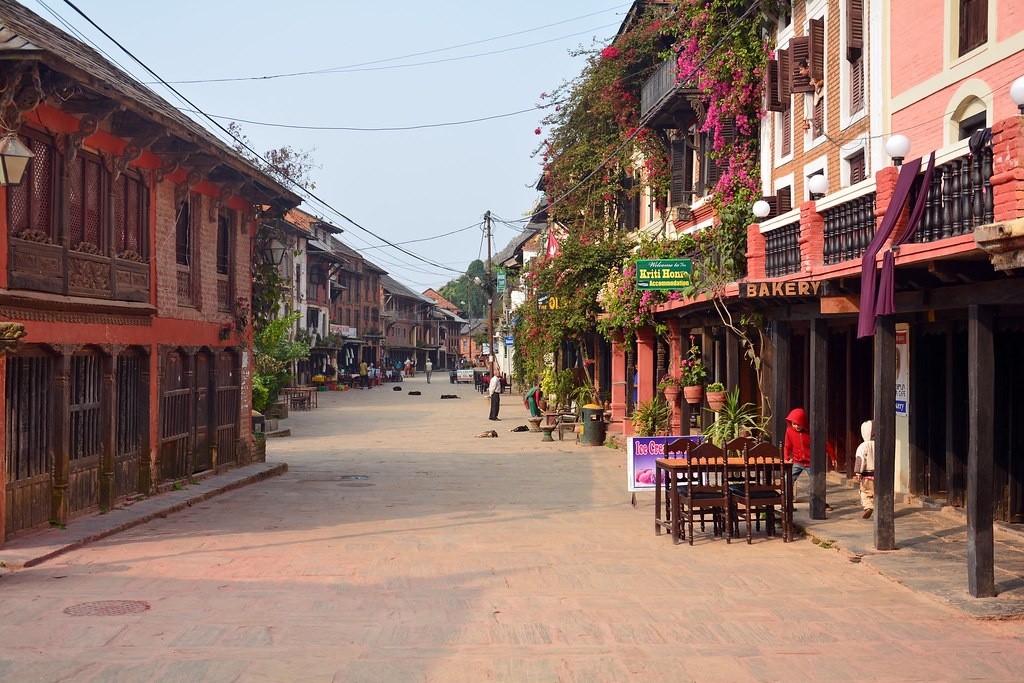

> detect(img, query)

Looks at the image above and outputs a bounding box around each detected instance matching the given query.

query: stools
[559,422,579,440]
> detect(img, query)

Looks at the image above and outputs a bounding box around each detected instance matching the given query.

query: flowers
[656,335,707,392]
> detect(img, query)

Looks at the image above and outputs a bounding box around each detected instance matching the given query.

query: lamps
[886,135,910,166]
[677,181,703,222]
[0,133,34,187]
[1011,77,1024,114]
[254,235,285,265]
[753,200,770,222]
[809,175,828,199]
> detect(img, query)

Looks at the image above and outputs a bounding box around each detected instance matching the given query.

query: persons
[360,357,416,389]
[633,361,638,411]
[489,369,501,421]
[423,358,433,384]
[784,408,837,511]
[854,420,874,519]
[524,383,542,417]
[463,356,467,365]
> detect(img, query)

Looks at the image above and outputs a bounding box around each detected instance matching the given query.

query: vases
[684,385,702,403]
[664,386,681,400]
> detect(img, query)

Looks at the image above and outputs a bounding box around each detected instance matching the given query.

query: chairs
[664,437,787,546]
[474,370,512,393]
[290,387,310,411]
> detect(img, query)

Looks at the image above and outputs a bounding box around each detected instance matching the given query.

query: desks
[655,457,794,545]
[284,387,318,409]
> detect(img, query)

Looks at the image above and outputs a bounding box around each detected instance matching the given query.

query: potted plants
[706,383,728,410]
[326,335,343,348]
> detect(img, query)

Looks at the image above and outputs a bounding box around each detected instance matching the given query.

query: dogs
[392,386,401,391]
[441,395,462,399]
[473,430,498,439]
[408,391,421,395]
[511,424,529,432]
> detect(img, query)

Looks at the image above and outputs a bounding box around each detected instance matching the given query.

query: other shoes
[826,503,832,511]
[494,418,501,421]
[863,508,873,519]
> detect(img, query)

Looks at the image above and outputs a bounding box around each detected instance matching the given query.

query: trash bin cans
[581,404,603,445]
[252,415,266,433]
